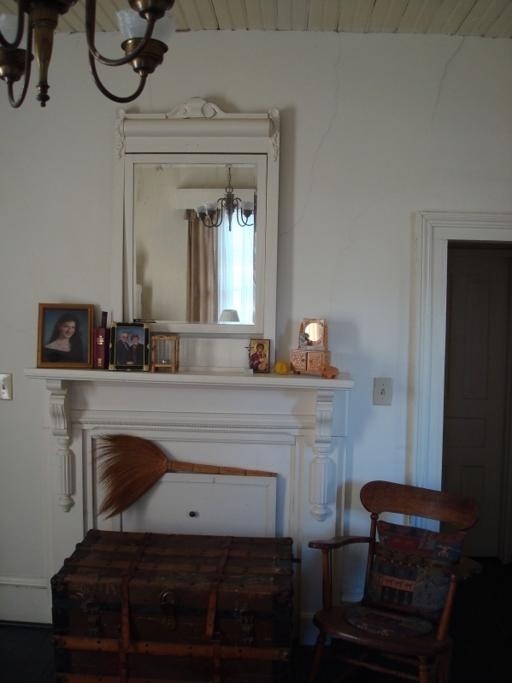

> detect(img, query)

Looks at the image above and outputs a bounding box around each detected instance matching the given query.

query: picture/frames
[150,333,179,373]
[108,320,151,373]
[249,339,271,373]
[36,302,95,369]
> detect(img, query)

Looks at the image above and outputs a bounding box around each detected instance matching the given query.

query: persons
[42,308,89,364]
[250,341,269,373]
[115,328,143,364]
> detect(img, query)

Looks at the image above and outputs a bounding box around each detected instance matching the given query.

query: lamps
[194,163,255,232]
[0,0,176,109]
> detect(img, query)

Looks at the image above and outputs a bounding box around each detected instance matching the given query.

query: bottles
[93,311,110,369]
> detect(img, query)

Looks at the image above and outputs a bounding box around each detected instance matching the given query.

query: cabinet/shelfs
[289,347,330,376]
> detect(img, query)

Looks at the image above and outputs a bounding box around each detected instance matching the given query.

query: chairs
[307,480,482,683]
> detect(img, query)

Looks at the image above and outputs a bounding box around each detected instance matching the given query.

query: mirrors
[298,318,327,350]
[106,98,282,369]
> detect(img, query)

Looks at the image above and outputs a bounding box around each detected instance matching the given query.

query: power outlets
[1,373,12,401]
[371,377,393,406]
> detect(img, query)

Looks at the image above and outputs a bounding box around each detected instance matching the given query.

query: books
[94,327,109,369]
